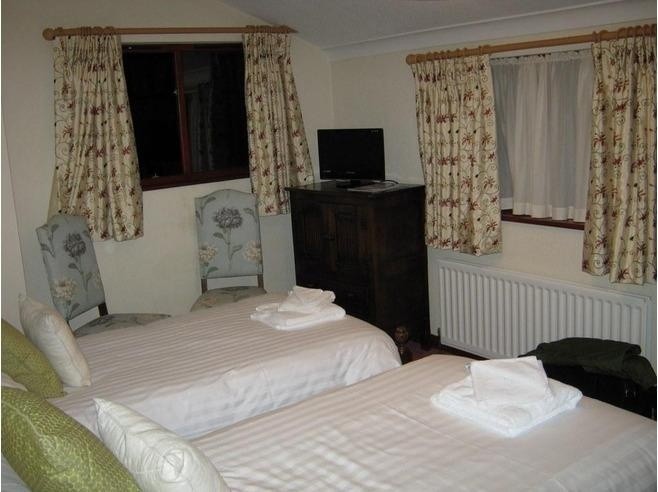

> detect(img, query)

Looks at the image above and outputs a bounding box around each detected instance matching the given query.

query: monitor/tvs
[317,128,385,188]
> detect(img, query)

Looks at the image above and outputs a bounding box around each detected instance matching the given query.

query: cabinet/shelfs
[283,178,429,356]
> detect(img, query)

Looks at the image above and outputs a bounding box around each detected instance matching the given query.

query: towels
[251,301,347,333]
[278,284,337,315]
[430,366,584,438]
[464,353,553,408]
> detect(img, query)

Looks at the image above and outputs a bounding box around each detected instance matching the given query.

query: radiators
[436,256,652,367]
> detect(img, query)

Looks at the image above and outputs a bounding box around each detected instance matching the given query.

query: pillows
[88,395,232,492]
[17,292,93,388]
[1,384,142,492]
[1,319,69,401]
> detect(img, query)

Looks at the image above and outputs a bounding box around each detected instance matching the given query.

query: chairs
[34,210,173,340]
[187,187,269,317]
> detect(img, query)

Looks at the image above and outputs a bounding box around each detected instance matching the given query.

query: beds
[1,353,656,492]
[1,283,404,455]
